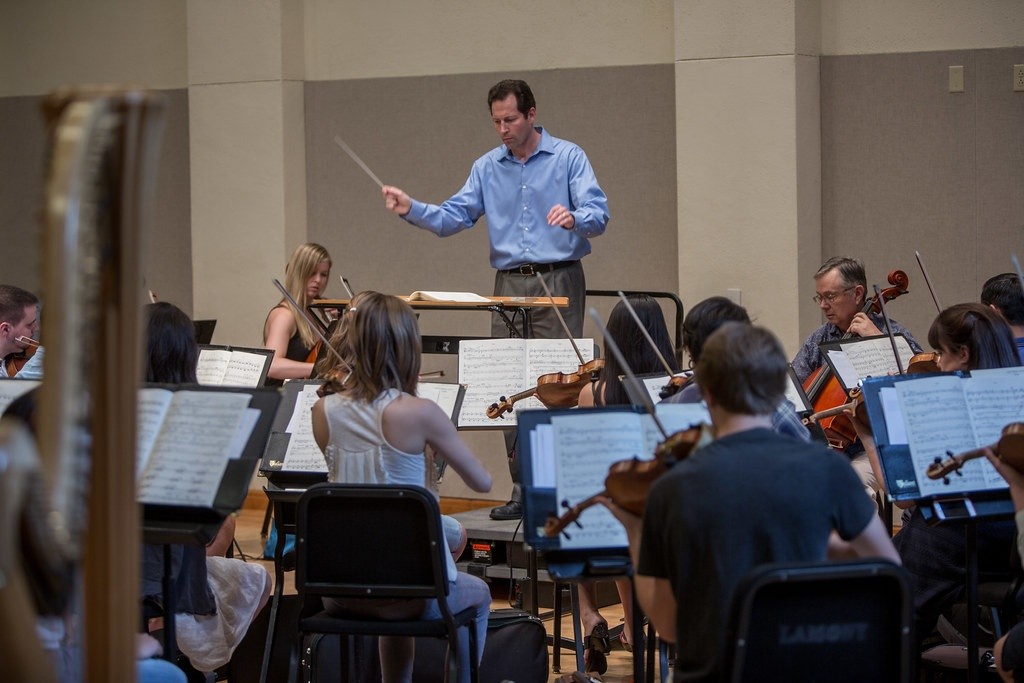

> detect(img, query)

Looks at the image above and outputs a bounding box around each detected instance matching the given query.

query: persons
[311,291,493,683]
[591,323,901,683]
[264,242,331,558]
[792,257,924,495]
[843,303,1021,644]
[0,285,273,682]
[980,273,1024,367]
[384,80,611,519]
[535,293,680,408]
[649,297,811,442]
[983,448,1024,683]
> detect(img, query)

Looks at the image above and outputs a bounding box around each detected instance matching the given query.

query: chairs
[286,482,482,683]
[663,556,921,682]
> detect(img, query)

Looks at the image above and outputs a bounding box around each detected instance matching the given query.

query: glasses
[812,288,856,306]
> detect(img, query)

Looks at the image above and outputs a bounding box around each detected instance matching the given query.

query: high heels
[620,626,647,653]
[583,623,611,675]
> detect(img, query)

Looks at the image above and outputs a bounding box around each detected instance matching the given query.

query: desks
[307,294,570,341]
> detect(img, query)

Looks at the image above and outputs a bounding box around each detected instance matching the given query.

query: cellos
[285,264,341,380]
[800,267,910,453]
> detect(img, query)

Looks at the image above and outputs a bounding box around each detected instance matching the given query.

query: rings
[563,214,566,218]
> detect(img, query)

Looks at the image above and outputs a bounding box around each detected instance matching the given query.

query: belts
[500,259,580,276]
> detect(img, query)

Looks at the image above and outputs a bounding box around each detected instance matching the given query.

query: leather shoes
[489,500,523,520]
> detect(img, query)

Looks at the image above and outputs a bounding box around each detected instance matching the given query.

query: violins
[926,421,1024,485]
[314,378,348,398]
[486,356,607,421]
[658,372,692,399]
[542,420,718,542]
[4,337,39,378]
[888,350,943,379]
[799,377,873,435]
